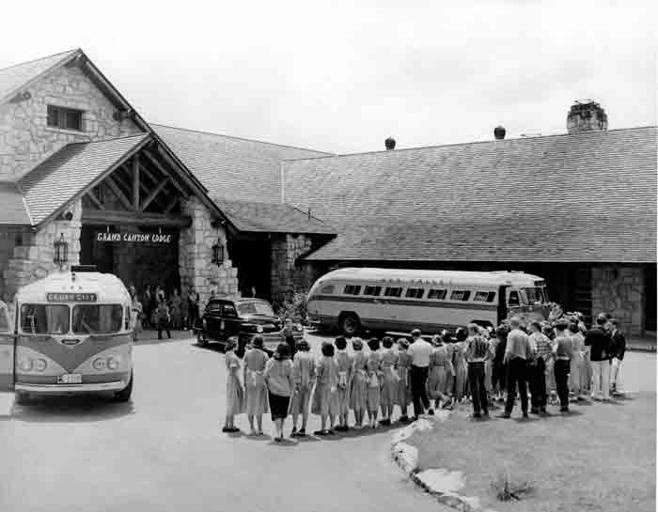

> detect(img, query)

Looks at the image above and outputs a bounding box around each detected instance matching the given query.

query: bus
[10,270,144,408]
[305,267,551,342]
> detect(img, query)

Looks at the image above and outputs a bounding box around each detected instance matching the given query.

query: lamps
[54,231,68,270]
[211,236,225,268]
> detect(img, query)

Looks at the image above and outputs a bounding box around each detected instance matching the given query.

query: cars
[191,294,305,359]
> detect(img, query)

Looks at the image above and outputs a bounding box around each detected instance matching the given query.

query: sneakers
[221,412,410,444]
[570,387,619,403]
[469,403,569,420]
[426,400,451,415]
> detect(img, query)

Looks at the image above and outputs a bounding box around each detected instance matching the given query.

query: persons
[223,318,436,445]
[429,312,624,423]
[128,286,200,340]
